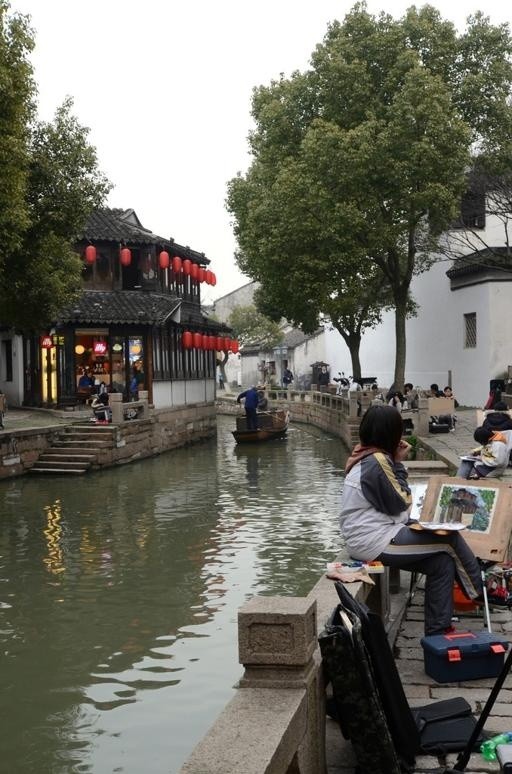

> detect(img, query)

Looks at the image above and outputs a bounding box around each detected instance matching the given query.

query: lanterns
[158,251,215,288]
[85,245,96,266]
[121,248,132,267]
[182,329,238,356]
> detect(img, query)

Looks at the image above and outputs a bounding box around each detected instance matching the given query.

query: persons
[317,364,330,392]
[218,371,226,389]
[340,405,512,636]
[257,391,270,411]
[454,426,510,479]
[282,366,293,389]
[79,368,113,425]
[483,401,512,467]
[237,386,260,431]
[347,377,459,433]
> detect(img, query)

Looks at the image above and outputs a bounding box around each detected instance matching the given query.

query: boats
[232,406,293,443]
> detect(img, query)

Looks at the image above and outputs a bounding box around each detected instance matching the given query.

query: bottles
[479,731,512,761]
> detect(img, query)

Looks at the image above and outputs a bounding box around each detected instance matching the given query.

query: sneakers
[470,592,509,610]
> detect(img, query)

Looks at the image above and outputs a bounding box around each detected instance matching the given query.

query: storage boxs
[418,628,512,682]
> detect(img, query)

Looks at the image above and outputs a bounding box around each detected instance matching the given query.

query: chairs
[328,580,492,772]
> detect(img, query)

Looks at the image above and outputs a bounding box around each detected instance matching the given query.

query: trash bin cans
[358,377,378,391]
